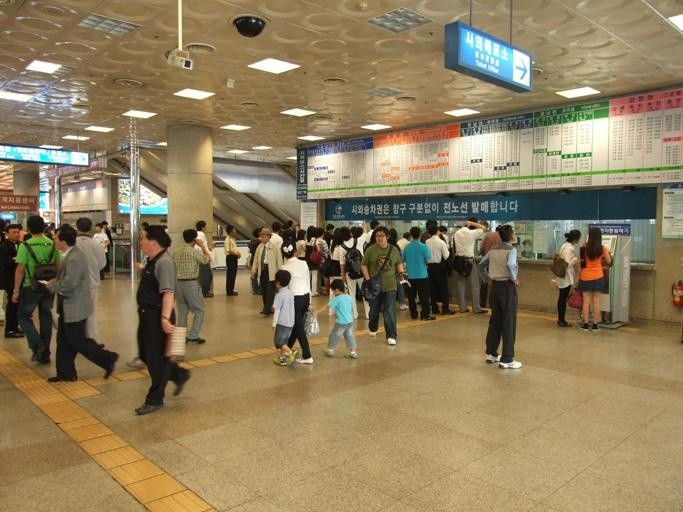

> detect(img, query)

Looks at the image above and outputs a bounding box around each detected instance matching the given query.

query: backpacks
[31,257,57,294]
[345,246,362,280]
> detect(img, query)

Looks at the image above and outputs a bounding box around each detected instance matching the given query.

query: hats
[257,228,272,235]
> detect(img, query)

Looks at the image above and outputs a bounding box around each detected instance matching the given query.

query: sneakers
[399,303,408,311]
[29,342,46,362]
[579,323,589,332]
[499,361,523,370]
[460,308,469,314]
[591,324,600,333]
[288,349,299,368]
[410,313,418,321]
[556,319,573,329]
[485,352,501,364]
[386,335,397,346]
[368,329,377,338]
[472,309,489,315]
[188,337,206,347]
[344,351,359,359]
[37,351,52,364]
[322,348,335,357]
[203,292,213,297]
[272,356,287,366]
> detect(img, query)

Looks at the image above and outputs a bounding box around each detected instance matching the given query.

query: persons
[553,230,581,327]
[247,216,503,321]
[224,226,241,296]
[578,228,612,331]
[0,214,118,383]
[363,226,406,346]
[478,224,524,370]
[126,222,150,371]
[172,228,212,344]
[314,279,360,359]
[195,220,214,299]
[136,226,191,415]
[279,238,314,364]
[272,270,298,366]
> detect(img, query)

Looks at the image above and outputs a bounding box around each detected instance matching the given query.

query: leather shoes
[442,309,455,316]
[296,357,314,364]
[48,372,77,384]
[131,402,165,414]
[420,313,434,321]
[227,291,238,296]
[103,351,120,380]
[3,327,24,339]
[173,369,193,395]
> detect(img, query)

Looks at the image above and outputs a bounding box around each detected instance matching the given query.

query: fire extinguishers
[671,280,683,308]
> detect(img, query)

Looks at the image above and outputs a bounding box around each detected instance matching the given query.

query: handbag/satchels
[452,255,474,278]
[310,250,322,265]
[566,288,583,310]
[361,273,384,303]
[550,254,567,279]
[302,307,319,338]
[320,259,340,278]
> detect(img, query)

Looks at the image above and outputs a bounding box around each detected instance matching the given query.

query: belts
[178,278,197,282]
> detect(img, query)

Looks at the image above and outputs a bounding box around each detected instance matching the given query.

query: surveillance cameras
[166,52,193,71]
[233,16,265,38]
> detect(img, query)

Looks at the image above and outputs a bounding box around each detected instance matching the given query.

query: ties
[260,246,266,271]
